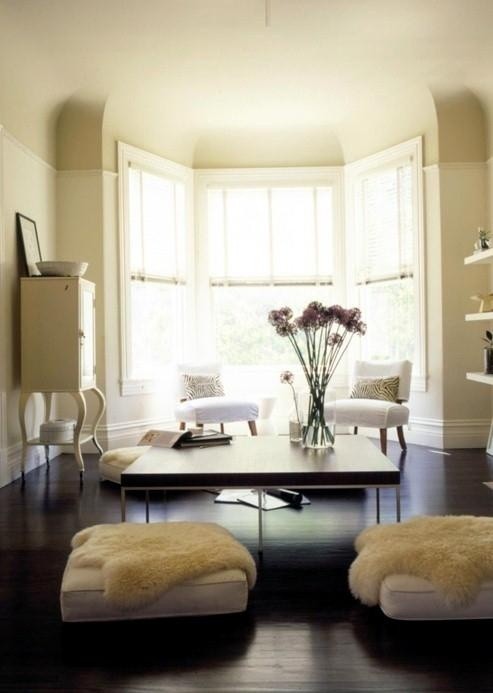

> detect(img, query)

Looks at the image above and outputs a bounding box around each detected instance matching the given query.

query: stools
[95,445,149,484]
[346,511,492,621]
[58,519,258,621]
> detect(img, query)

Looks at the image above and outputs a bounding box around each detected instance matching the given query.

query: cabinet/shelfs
[463,245,493,388]
[16,276,107,474]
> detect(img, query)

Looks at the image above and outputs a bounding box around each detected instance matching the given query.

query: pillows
[349,375,400,401]
[181,372,224,399]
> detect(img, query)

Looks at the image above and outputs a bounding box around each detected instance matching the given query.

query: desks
[117,433,403,527]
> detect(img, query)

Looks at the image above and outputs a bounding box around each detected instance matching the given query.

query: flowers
[266,299,367,445]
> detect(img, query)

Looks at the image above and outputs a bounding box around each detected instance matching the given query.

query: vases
[302,386,339,449]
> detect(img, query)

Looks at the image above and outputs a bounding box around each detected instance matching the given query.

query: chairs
[323,357,412,454]
[172,362,259,434]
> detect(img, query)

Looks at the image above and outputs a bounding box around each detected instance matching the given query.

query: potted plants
[480,329,493,375]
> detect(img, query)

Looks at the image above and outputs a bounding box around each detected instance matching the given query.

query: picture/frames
[15,210,42,276]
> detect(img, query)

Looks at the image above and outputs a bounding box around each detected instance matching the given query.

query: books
[214,489,311,511]
[136,429,232,448]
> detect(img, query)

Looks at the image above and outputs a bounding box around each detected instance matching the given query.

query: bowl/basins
[35,260,90,277]
